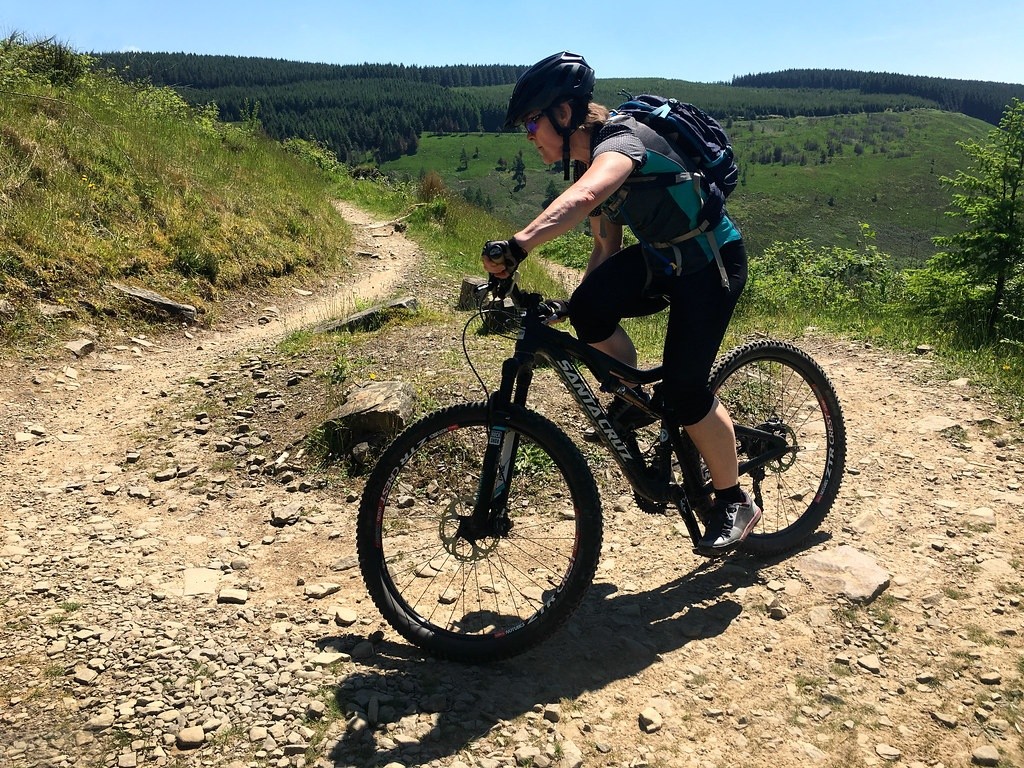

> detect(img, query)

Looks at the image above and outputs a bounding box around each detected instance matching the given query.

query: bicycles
[356,244,847,663]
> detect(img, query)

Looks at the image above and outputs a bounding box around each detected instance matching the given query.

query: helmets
[503,51,596,132]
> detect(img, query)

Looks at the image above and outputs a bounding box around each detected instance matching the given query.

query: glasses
[524,111,544,135]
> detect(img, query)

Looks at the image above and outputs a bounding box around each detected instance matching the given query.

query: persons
[481,51,761,555]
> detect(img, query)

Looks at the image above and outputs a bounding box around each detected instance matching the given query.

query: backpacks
[600,88,739,296]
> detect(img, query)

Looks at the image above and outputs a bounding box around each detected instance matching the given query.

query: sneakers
[697,492,762,551]
[582,386,650,444]
